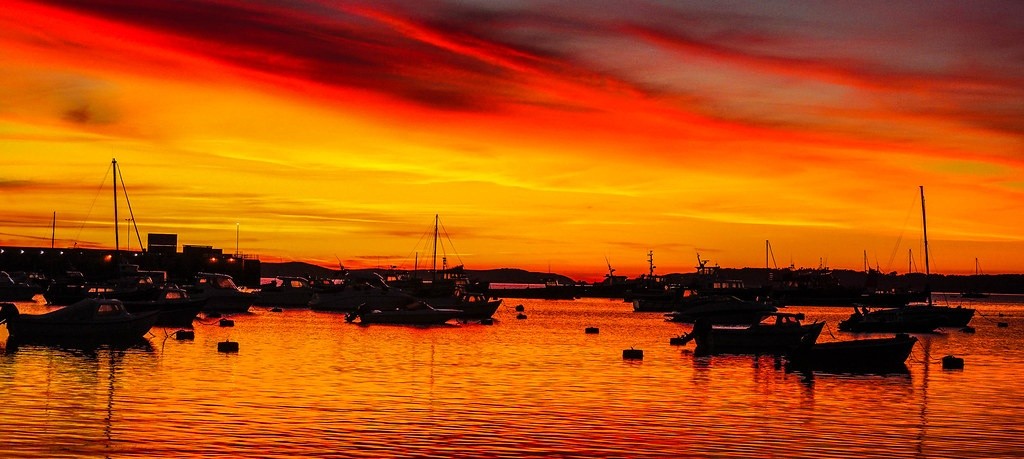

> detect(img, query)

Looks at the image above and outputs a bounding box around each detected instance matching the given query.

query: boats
[521,278,586,300]
[592,183,976,374]
[0,158,504,343]
